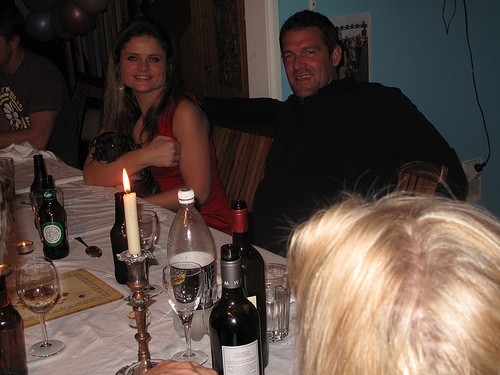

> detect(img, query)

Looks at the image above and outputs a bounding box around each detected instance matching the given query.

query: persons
[284,189,500,375]
[0,0,86,172]
[177,8,469,261]
[82,16,236,236]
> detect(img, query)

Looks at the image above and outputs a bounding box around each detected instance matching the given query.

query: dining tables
[1,143,301,374]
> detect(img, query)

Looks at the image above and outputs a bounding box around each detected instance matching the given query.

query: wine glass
[137,209,165,298]
[161,261,210,366]
[16,256,65,357]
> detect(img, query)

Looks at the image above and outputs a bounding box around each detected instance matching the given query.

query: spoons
[74,237,103,258]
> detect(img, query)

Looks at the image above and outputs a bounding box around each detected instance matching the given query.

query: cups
[264,261,290,343]
[0,156,16,203]
[125,358,165,375]
[29,186,64,244]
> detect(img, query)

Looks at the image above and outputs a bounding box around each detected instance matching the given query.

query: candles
[121,165,142,258]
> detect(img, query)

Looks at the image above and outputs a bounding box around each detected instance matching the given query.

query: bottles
[209,242,264,375]
[29,153,50,229]
[0,276,29,375]
[229,198,269,367]
[109,192,130,283]
[166,188,217,341]
[38,174,70,260]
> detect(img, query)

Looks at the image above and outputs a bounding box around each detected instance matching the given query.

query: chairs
[74,71,106,171]
[211,124,274,215]
[396,163,439,196]
